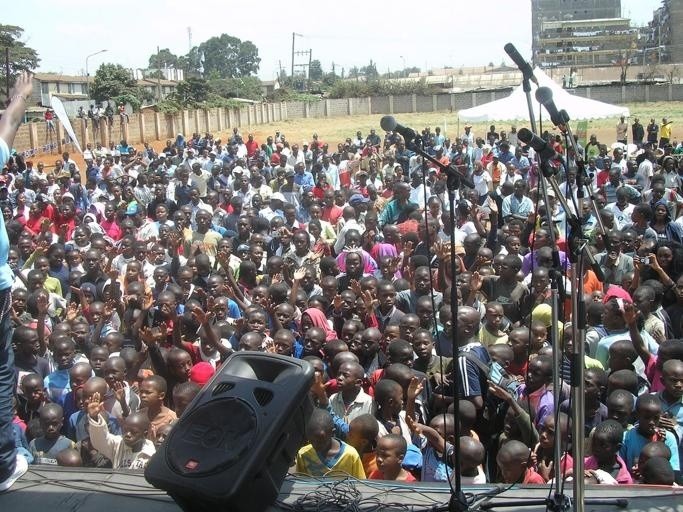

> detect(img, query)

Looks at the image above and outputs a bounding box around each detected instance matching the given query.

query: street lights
[85,49,108,101]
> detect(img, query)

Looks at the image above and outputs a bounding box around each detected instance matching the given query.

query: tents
[457,68,629,145]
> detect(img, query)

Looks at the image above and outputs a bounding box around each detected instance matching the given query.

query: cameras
[639,257,649,264]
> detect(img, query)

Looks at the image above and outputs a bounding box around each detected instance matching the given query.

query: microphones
[535,86,566,133]
[504,43,537,83]
[517,128,562,160]
[380,116,417,139]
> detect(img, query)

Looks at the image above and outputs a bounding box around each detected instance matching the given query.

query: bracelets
[12,95,26,102]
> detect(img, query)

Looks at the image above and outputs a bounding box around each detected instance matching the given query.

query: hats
[464,125,472,129]
[80,282,97,299]
[62,193,75,201]
[348,194,370,206]
[125,204,143,215]
[233,166,243,174]
[270,193,289,203]
[457,199,472,213]
[532,304,553,329]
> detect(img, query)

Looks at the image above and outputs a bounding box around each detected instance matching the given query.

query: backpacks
[458,342,517,434]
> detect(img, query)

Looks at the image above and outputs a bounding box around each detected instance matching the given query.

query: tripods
[415,189,505,512]
[480,281,627,512]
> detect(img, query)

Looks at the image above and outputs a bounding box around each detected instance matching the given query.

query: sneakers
[1,454,28,491]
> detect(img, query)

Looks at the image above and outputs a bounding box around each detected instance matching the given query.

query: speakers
[144,351,316,512]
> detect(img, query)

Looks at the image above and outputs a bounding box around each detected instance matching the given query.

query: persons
[45,101,129,133]
[0,128,683,485]
[1,69,34,491]
